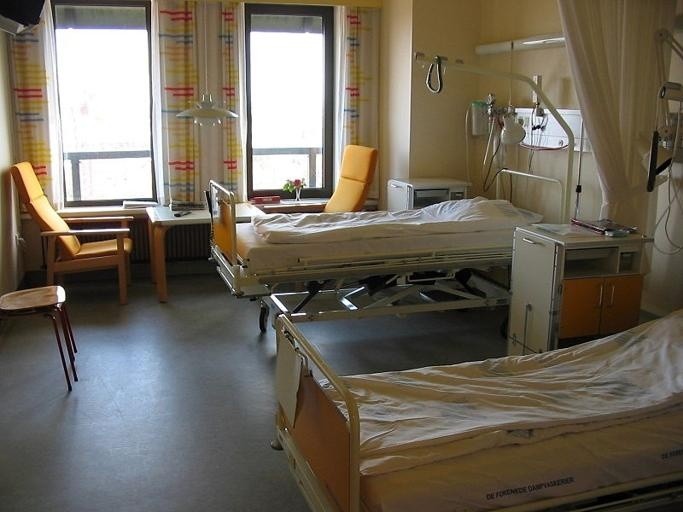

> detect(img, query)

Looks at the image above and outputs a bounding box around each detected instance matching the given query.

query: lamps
[175,0,239,129]
[500,111,526,147]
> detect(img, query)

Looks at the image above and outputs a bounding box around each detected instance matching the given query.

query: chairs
[323,145,377,212]
[11,162,136,305]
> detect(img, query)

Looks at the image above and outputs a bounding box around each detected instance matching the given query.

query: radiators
[40,222,211,268]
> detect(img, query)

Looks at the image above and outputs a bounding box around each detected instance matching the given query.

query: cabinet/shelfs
[507,223,655,356]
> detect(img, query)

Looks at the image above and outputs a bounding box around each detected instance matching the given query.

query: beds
[207,168,563,322]
[272,311,683,511]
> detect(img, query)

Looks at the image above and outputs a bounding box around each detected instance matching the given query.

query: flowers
[283,175,307,192]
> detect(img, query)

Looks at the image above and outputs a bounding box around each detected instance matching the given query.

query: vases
[296,188,301,201]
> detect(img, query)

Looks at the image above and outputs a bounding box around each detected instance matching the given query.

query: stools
[0,285,78,392]
